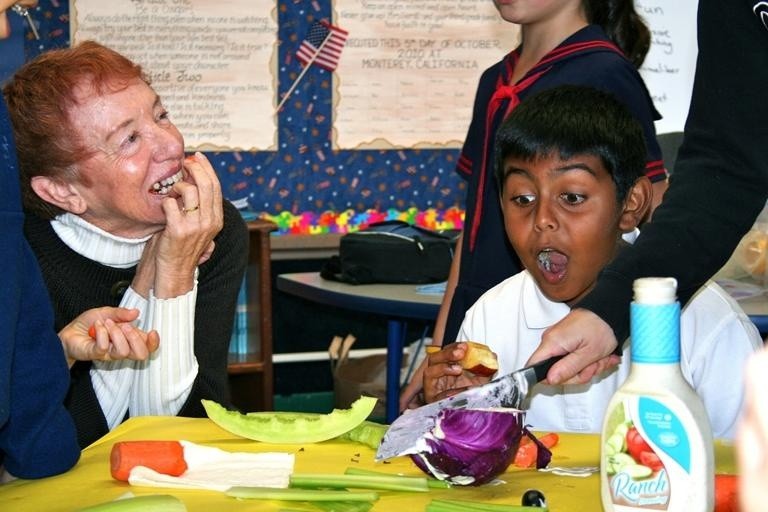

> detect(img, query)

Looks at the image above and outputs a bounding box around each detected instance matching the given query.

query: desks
[277,272,451,423]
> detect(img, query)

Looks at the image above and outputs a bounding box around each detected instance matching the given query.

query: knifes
[370,346,624,465]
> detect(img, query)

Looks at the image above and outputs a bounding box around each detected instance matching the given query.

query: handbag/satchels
[321,327,420,416]
[317,218,456,286]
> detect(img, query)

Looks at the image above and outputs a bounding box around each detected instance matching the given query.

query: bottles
[597,274,716,512]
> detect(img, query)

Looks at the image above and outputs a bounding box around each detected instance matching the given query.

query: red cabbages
[404,406,553,487]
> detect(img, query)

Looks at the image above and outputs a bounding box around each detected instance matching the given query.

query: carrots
[110,440,188,483]
[514,431,559,468]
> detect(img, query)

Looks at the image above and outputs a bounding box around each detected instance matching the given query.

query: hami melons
[201,394,378,443]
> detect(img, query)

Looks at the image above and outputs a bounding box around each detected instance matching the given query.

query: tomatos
[627,428,663,472]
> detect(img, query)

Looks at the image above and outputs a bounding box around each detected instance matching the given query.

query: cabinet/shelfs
[225,221,277,412]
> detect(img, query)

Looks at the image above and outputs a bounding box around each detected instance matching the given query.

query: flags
[294,18,351,75]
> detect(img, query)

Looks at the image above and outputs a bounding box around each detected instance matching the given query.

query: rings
[181,204,199,215]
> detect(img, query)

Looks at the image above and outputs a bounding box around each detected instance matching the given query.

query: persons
[0,1,162,490]
[0,39,253,454]
[521,1,767,389]
[393,1,676,415]
[417,83,768,448]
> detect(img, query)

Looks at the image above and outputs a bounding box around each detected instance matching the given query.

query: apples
[458,341,499,377]
